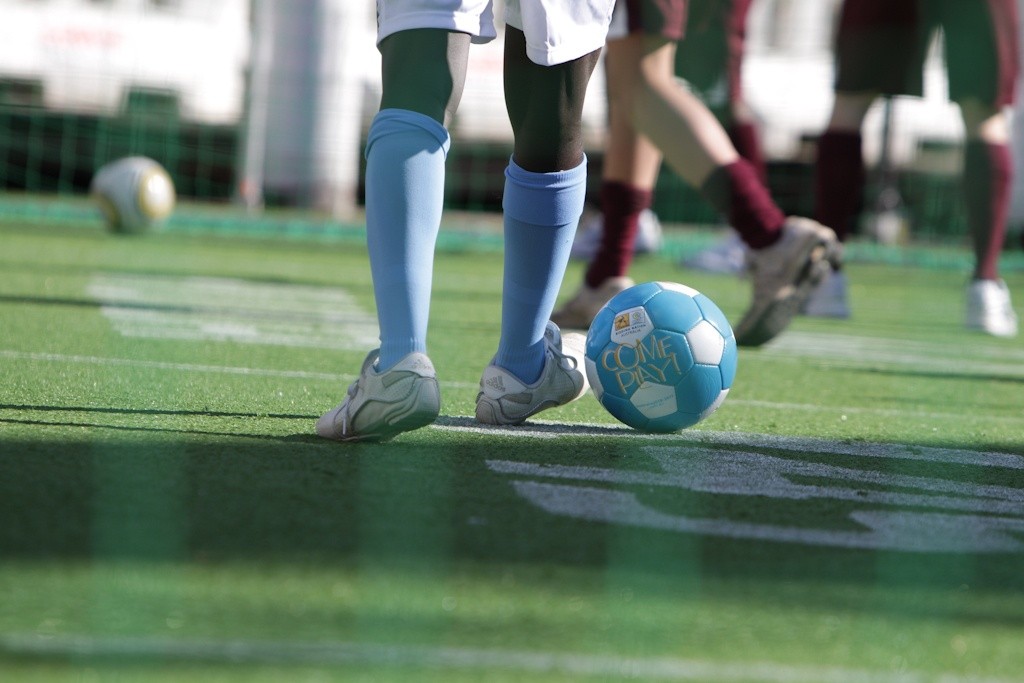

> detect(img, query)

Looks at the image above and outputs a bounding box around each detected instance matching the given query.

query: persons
[315,0,591,446]
[780,0,1024,341]
[548,0,835,349]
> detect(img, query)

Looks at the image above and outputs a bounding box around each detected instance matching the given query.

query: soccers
[584,278,738,434]
[92,157,175,234]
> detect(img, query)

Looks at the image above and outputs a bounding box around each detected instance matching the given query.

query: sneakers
[550,277,636,329]
[475,319,592,424]
[732,215,838,348]
[316,346,441,441]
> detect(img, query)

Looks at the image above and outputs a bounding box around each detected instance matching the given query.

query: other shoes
[681,229,751,278]
[964,278,1019,337]
[569,208,661,258]
[802,266,848,317]
